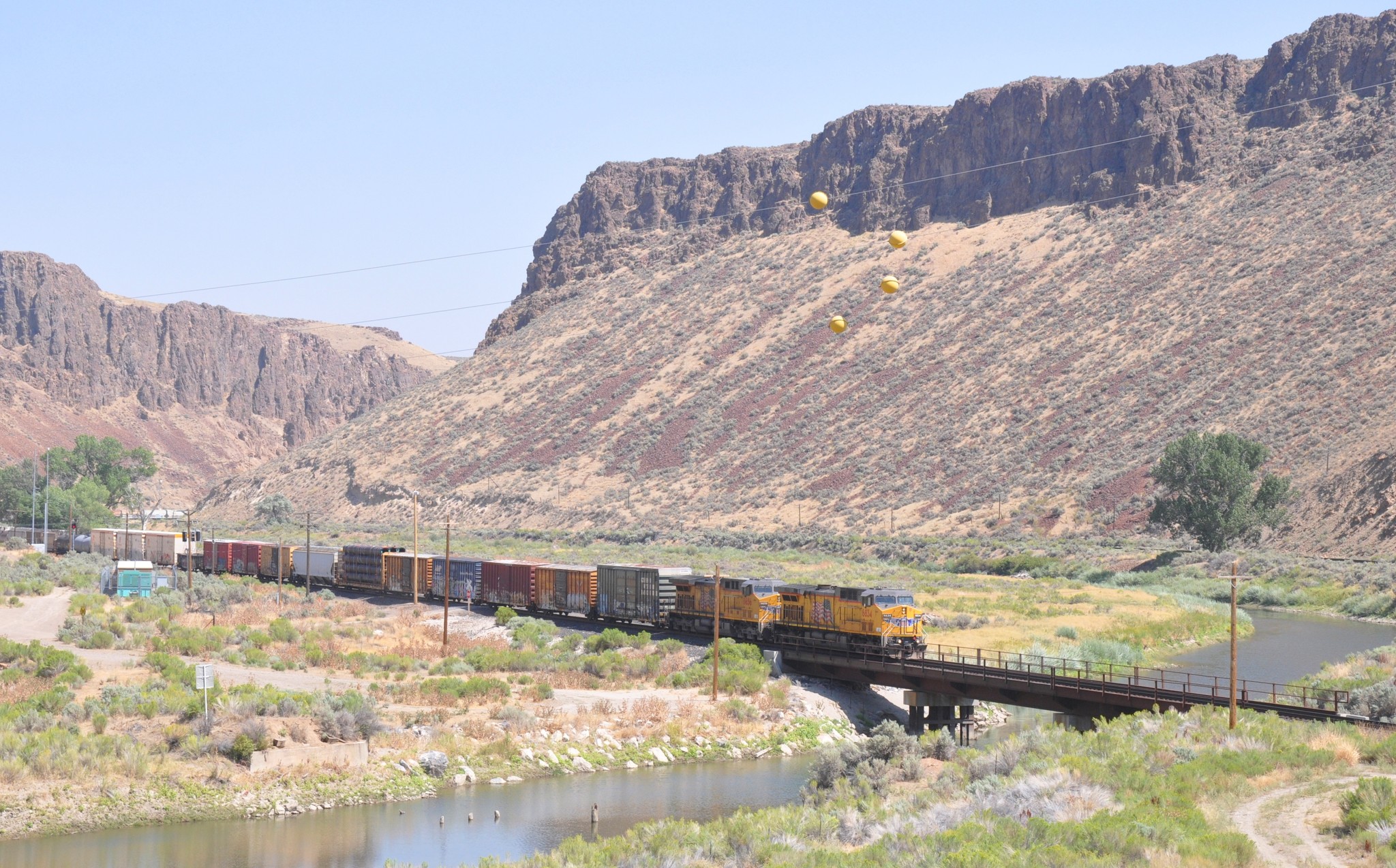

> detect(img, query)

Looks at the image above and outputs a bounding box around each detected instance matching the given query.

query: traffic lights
[71,523,76,529]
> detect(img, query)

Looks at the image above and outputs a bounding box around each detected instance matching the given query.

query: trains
[1,525,928,663]
